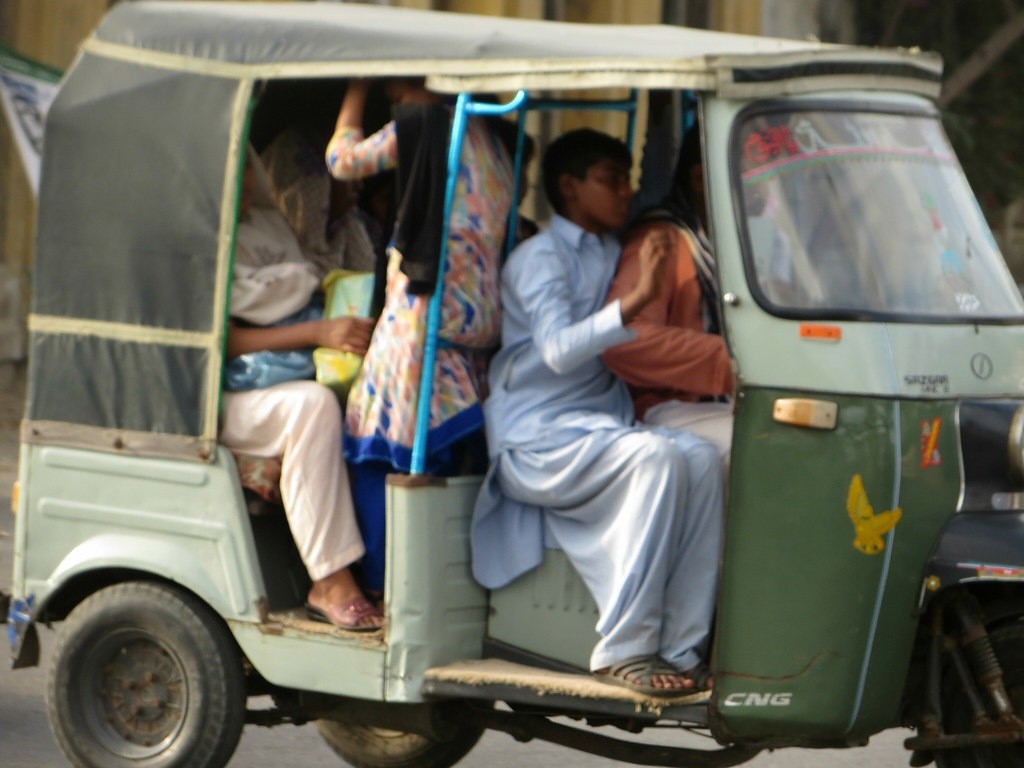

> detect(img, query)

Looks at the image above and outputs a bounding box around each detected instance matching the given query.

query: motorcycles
[8,0,1024,768]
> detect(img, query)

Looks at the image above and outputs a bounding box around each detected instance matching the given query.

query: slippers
[305,600,383,630]
[683,661,713,690]
[593,653,696,695]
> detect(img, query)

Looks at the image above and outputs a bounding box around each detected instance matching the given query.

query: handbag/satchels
[311,270,378,404]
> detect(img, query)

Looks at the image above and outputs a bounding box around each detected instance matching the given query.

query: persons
[216,76,538,630]
[469,117,767,693]
[959,196,1024,447]
